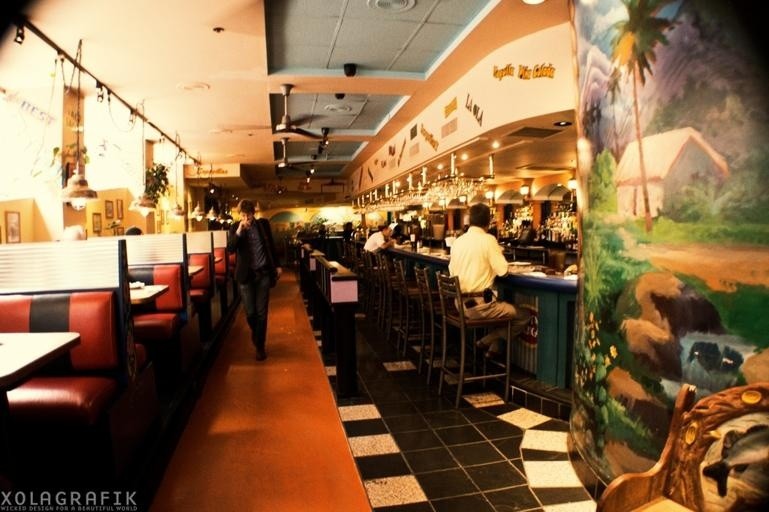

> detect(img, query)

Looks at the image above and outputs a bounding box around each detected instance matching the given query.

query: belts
[462,293,483,297]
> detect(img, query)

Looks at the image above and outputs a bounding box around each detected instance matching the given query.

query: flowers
[141,164,171,200]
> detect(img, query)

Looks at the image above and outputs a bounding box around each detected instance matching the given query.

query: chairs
[338,239,478,384]
[435,271,515,409]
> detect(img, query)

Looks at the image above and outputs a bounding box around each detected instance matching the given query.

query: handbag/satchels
[269,260,276,289]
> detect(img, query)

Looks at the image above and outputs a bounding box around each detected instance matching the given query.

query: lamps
[129,100,158,217]
[168,132,236,225]
[53,38,100,212]
[347,150,578,209]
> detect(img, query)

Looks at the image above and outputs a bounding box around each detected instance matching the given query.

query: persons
[126,225,144,235]
[228,198,282,360]
[448,204,532,359]
[343,222,355,241]
[360,226,398,258]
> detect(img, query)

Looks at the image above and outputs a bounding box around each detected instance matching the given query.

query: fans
[221,83,327,140]
[252,137,318,173]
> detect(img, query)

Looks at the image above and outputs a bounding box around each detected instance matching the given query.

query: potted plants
[140,193,156,202]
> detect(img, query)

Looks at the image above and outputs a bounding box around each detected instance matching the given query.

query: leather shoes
[484,351,506,362]
[255,350,266,361]
[477,342,487,352]
